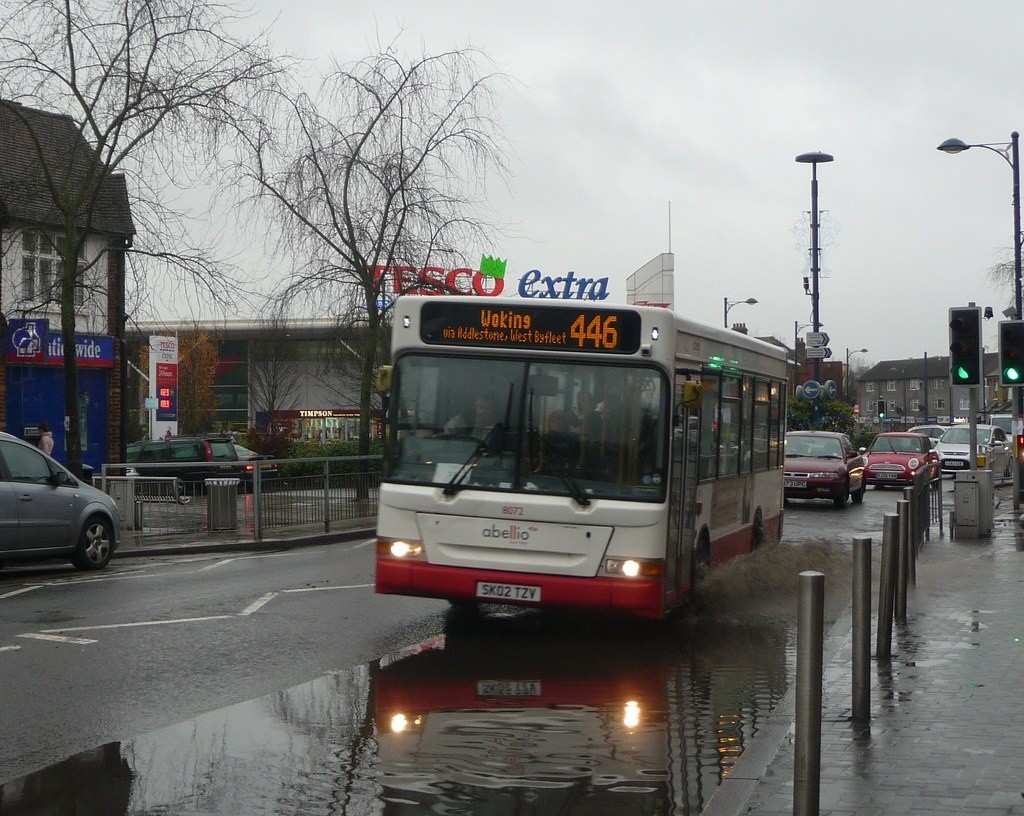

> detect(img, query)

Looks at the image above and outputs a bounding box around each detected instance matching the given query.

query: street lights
[847,348,868,401]
[794,152,834,420]
[937,138,1022,511]
[794,323,824,398]
[724,297,759,328]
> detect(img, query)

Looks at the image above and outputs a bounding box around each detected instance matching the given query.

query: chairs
[908,439,920,449]
[822,441,839,455]
[809,446,824,457]
[791,441,808,455]
[976,433,987,443]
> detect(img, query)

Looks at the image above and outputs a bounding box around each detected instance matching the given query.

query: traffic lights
[947,306,983,388]
[877,400,884,418]
[998,320,1024,387]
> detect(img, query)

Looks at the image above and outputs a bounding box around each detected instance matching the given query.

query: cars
[859,432,941,490]
[0,431,120,572]
[989,414,1013,442]
[933,424,1013,481]
[908,424,949,449]
[233,444,282,494]
[784,432,868,510]
[126,436,240,504]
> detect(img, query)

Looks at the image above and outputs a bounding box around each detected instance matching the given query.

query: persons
[429,390,516,460]
[549,389,659,482]
[220,430,239,444]
[37,422,55,457]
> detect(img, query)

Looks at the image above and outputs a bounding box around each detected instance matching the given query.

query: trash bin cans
[203,478,240,530]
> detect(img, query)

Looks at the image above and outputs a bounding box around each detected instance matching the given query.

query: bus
[375,293,789,617]
